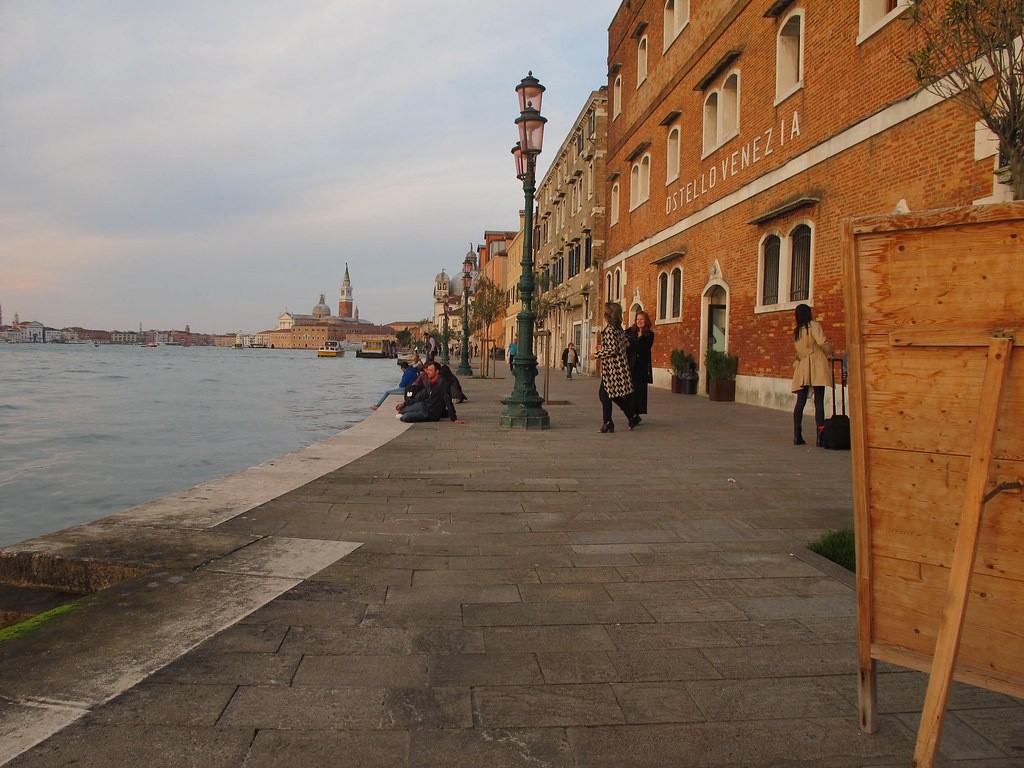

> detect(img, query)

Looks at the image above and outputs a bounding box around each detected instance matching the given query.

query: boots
[816,430,821,447]
[794,429,805,445]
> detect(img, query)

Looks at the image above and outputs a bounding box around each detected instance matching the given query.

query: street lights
[440,293,452,366]
[455,259,473,378]
[498,70,551,430]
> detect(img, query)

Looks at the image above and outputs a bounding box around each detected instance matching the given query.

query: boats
[317,340,345,358]
[249,344,269,348]
[140,342,158,347]
[356,339,399,359]
[397,346,418,366]
[232,343,243,350]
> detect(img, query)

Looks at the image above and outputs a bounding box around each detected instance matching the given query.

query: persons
[790,304,835,447]
[561,342,581,380]
[370,333,496,424]
[507,336,518,372]
[624,311,655,425]
[590,302,641,433]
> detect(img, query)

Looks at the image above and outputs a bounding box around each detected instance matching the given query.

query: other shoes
[567,376,572,380]
[395,414,403,420]
[370,405,378,410]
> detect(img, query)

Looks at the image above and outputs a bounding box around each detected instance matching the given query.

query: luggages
[820,358,850,450]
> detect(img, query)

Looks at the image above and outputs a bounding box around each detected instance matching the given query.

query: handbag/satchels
[560,362,564,371]
[576,363,581,374]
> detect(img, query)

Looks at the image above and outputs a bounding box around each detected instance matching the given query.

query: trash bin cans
[679,362,696,394]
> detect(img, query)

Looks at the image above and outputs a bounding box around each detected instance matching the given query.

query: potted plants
[667,349,699,394]
[495,348,505,360]
[704,350,739,401]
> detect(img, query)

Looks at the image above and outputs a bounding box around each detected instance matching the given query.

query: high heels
[598,422,614,433]
[628,415,641,430]
